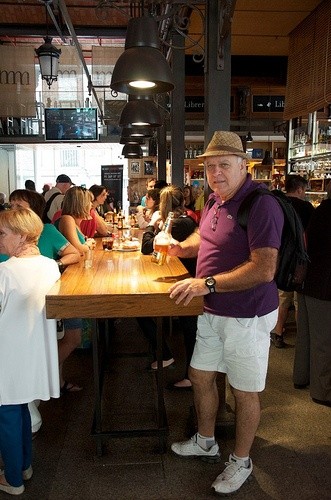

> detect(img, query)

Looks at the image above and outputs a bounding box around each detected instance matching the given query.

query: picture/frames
[128,159,157,178]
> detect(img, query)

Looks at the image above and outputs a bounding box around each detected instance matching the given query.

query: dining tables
[46,218,204,456]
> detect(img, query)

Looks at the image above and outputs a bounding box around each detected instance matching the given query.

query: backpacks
[238,190,307,293]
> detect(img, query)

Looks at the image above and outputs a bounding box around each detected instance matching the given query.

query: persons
[271,174,315,348]
[153,130,285,494]
[0,173,204,440]
[0,207,61,495]
[292,183,331,407]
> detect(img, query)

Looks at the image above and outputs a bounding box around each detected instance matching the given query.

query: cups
[78,249,93,269]
[131,228,138,237]
[101,237,114,251]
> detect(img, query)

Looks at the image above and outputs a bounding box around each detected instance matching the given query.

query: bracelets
[57,260,62,266]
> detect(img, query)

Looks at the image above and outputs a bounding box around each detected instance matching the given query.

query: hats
[196,130,248,162]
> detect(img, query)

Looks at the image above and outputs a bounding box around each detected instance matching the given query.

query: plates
[114,246,142,251]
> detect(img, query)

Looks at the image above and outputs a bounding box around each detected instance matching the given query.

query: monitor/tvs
[44,108,99,142]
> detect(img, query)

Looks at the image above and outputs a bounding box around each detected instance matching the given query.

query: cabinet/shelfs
[159,139,286,195]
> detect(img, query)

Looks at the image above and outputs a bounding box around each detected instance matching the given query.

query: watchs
[205,277,216,292]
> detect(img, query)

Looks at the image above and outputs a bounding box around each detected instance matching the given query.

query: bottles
[118,213,123,229]
[151,211,174,266]
[167,144,201,159]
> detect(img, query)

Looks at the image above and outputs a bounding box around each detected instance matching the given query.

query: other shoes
[312,398,331,407]
[167,380,192,391]
[294,382,310,388]
[60,379,87,392]
[271,333,285,348]
[151,355,173,369]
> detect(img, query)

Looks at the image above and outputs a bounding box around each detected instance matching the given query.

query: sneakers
[211,454,253,496]
[0,469,25,495]
[23,465,34,480]
[170,434,220,456]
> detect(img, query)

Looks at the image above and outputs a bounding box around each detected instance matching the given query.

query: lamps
[33,0,61,89]
[93,0,207,158]
